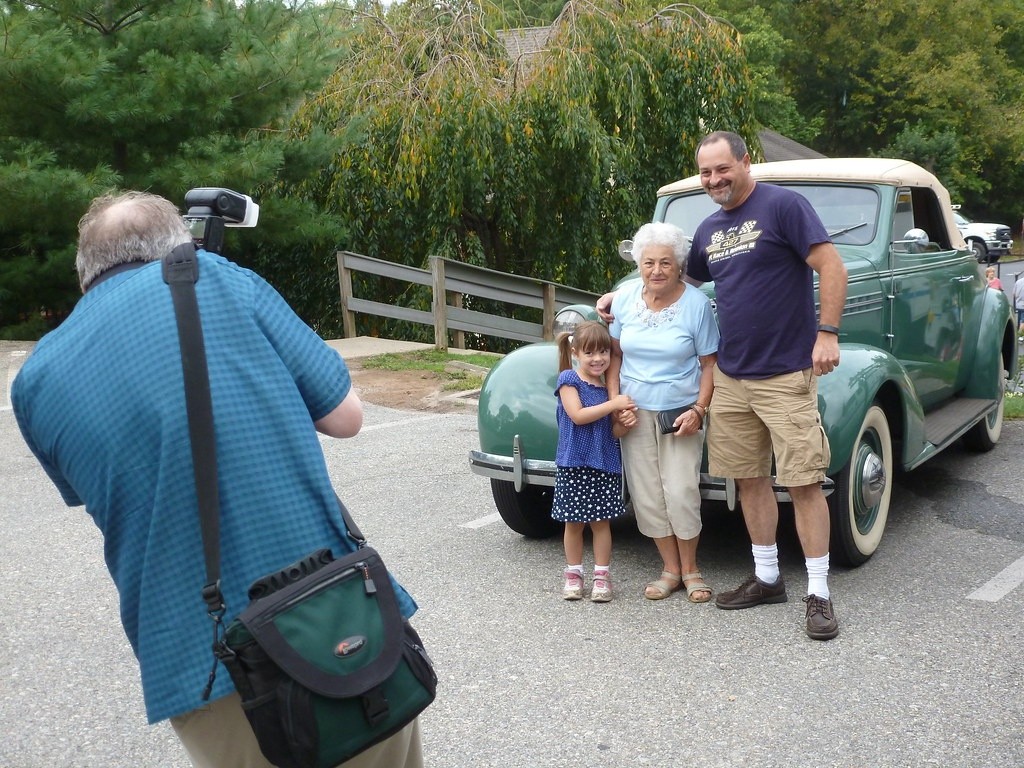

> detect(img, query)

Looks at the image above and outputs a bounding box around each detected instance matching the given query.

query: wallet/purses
[656,407,702,435]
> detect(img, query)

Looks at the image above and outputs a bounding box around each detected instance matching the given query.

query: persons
[985,267,1003,293]
[552,323,638,602]
[1012,273,1023,341]
[606,222,720,605]
[12,185,424,768]
[597,132,846,641]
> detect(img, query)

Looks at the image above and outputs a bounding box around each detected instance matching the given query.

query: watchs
[695,403,710,414]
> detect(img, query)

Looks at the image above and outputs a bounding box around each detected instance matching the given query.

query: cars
[468,157,1018,571]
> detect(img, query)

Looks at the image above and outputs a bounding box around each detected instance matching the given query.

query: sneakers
[591,570,613,602]
[563,569,584,600]
[805,595,839,641]
[714,575,788,610]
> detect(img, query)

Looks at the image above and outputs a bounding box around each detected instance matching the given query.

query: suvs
[945,207,1012,264]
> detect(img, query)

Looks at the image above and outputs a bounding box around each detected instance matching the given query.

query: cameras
[181,186,260,258]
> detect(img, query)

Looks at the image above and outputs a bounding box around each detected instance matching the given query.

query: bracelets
[817,324,840,337]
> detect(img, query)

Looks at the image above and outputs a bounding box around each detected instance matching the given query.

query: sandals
[644,571,682,600]
[682,572,713,603]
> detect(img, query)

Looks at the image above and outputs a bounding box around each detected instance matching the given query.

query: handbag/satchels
[219,547,439,768]
